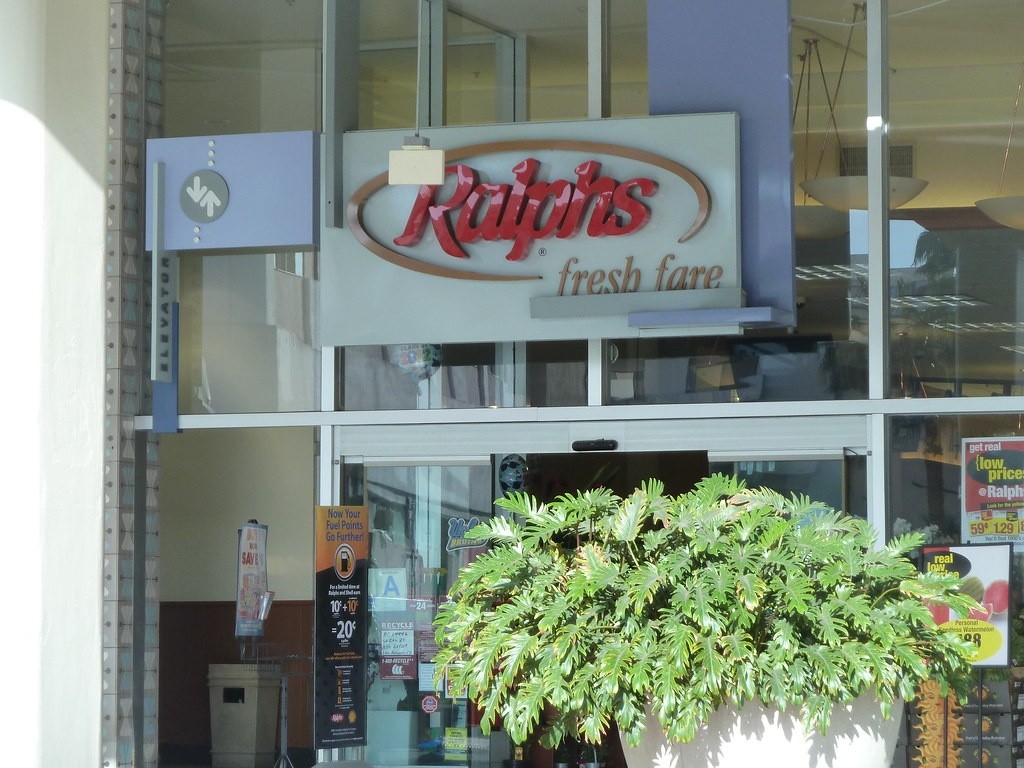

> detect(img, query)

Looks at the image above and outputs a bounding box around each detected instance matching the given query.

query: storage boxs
[905,667,1024,768]
[444,727,468,761]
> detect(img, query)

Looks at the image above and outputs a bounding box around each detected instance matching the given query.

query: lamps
[387,0,444,186]
[975,62,1024,231]
[793,38,849,241]
[798,0,929,214]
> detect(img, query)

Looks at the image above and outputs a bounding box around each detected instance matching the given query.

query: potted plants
[430,473,990,768]
[554,741,572,768]
[583,739,608,768]
